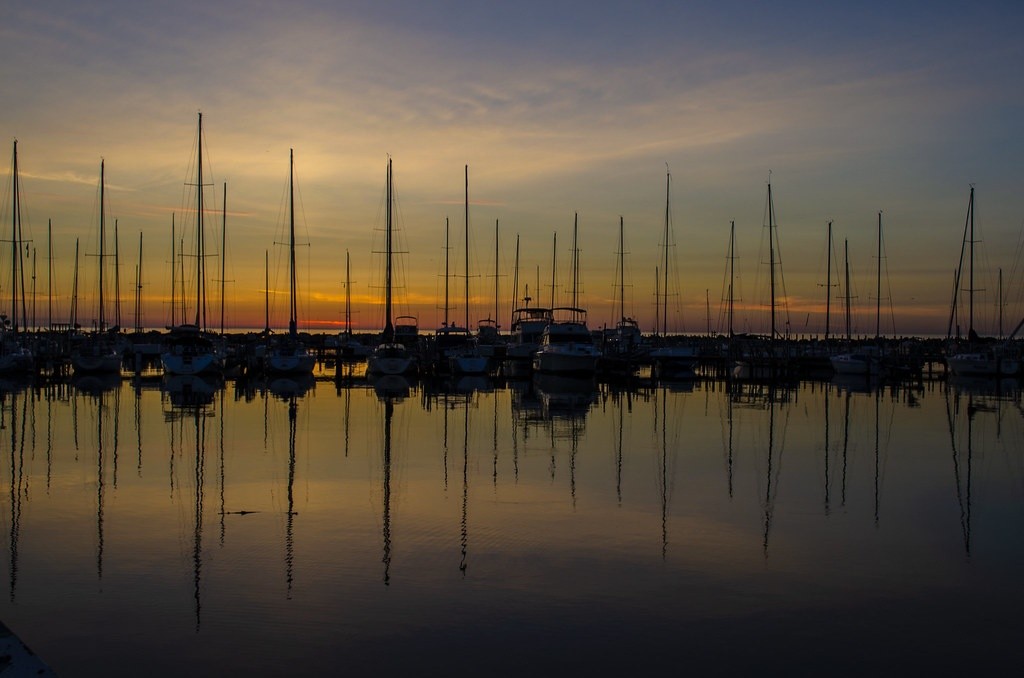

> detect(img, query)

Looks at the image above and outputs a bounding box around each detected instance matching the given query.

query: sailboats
[263,372,316,602]
[420,374,487,578]
[162,370,225,634]
[0,111,1024,379]
[366,368,418,583]
